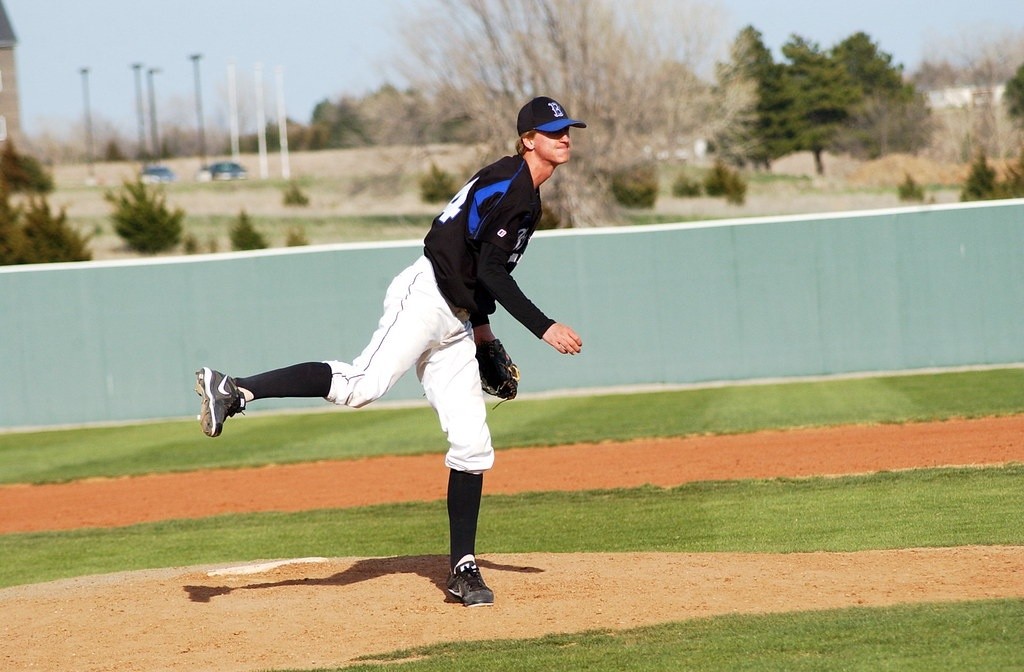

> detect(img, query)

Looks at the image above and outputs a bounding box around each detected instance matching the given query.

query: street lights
[79,68,93,177]
[143,69,160,165]
[131,62,150,167]
[190,55,208,169]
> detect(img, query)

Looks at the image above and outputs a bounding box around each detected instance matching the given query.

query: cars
[138,165,177,187]
[204,161,248,183]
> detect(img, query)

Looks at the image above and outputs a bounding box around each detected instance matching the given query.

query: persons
[193,97,587,608]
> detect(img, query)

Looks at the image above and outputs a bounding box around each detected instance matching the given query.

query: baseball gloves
[476,339,520,400]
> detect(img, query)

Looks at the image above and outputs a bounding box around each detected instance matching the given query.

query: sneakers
[445,565,493,608]
[196,366,248,438]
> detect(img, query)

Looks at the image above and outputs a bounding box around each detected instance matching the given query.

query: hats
[517,96,587,136]
